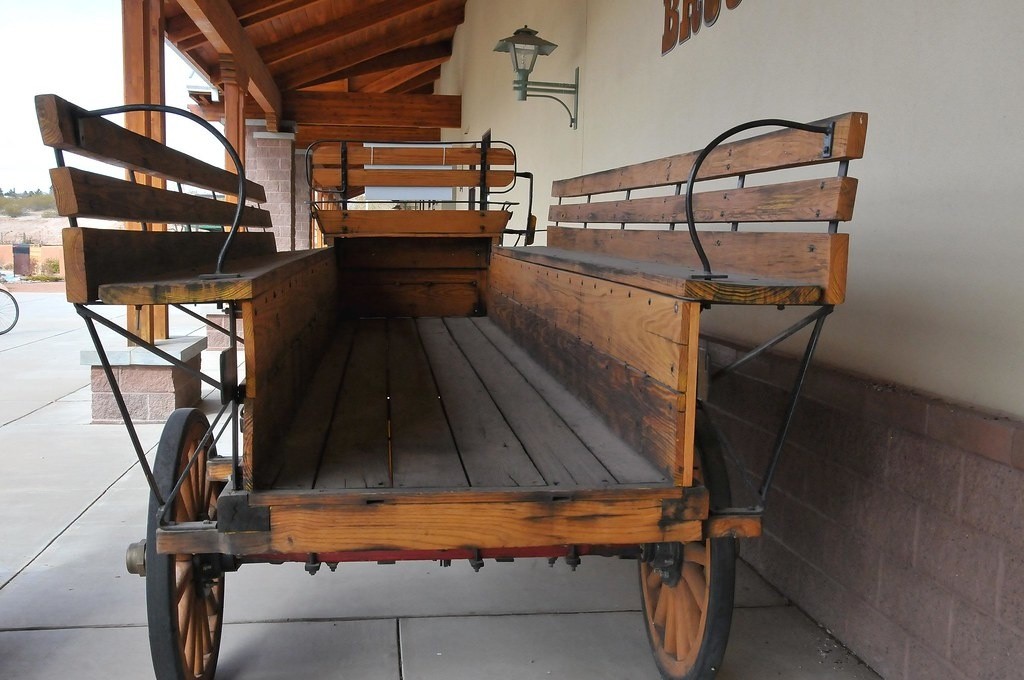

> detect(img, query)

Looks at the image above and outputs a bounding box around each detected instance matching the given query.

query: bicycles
[0,288,19,335]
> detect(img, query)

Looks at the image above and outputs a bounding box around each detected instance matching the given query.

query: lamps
[494,24,580,130]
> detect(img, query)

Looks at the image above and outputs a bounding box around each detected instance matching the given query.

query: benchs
[306,140,516,238]
[35,94,335,307]
[494,110,868,315]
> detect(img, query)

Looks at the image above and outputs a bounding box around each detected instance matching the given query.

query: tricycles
[35,95,870,680]
